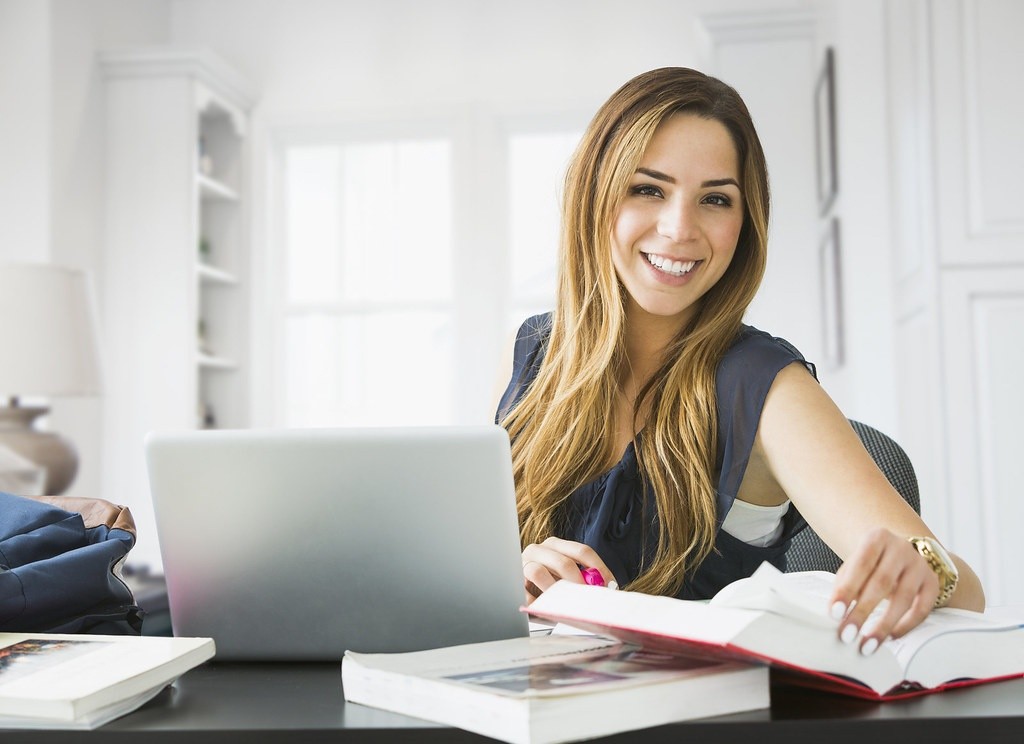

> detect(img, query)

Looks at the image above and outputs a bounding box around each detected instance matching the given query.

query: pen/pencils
[581,566,608,587]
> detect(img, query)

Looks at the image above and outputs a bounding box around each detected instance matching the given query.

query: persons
[496,67,985,657]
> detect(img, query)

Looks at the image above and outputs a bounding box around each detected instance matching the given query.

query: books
[520,559,1024,701]
[341,632,770,744]
[0,633,216,731]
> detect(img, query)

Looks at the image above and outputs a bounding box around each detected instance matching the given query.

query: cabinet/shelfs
[99,56,253,571]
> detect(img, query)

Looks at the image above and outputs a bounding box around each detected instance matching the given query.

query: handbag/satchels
[0,490,149,636]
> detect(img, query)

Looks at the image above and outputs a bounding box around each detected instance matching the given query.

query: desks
[0,612,1024,744]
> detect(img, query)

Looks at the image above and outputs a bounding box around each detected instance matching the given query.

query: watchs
[909,538,958,608]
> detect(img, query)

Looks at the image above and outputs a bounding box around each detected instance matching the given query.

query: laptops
[145,423,550,660]
